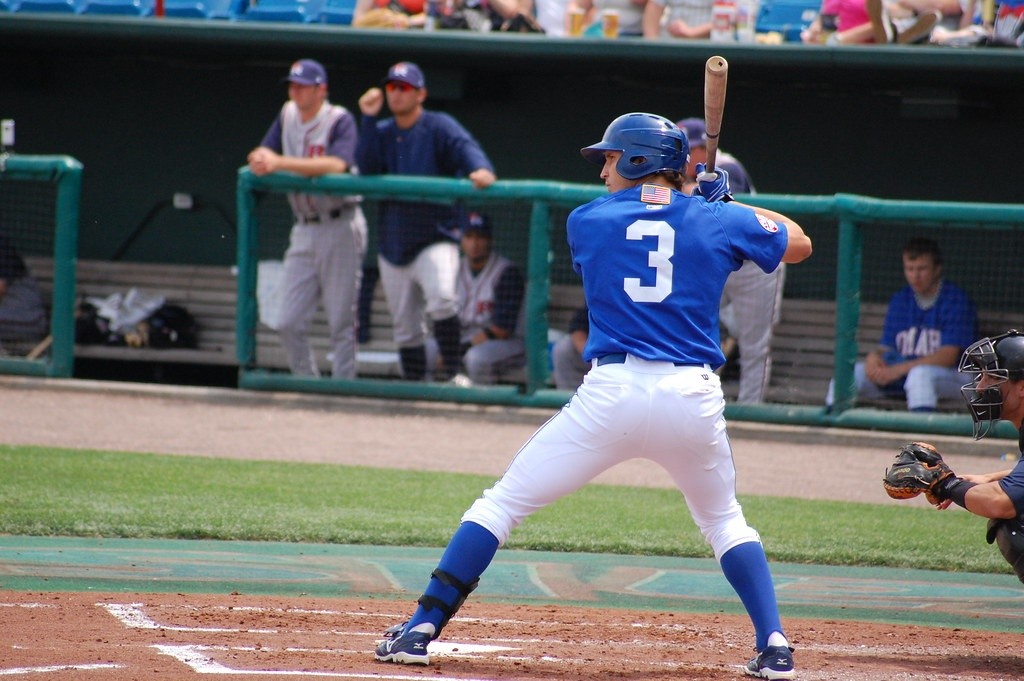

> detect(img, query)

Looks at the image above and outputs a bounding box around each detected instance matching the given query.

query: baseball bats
[703,55,730,173]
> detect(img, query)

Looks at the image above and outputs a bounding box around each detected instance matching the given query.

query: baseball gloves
[883,440,956,508]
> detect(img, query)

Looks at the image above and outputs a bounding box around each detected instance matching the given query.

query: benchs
[0,255,1024,414]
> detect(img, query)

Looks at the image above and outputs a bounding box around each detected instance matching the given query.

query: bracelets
[460,341,471,358]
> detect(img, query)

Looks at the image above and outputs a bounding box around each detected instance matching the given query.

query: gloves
[695,162,734,202]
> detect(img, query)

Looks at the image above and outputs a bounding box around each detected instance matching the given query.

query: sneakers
[373,623,430,664]
[744,645,795,681]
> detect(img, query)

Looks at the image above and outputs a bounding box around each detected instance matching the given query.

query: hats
[385,63,426,86]
[284,60,326,84]
[464,212,492,236]
[673,118,707,146]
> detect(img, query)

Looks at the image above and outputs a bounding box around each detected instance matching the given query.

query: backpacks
[74,293,198,350]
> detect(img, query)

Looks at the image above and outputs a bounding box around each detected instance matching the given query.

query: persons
[247,60,368,382]
[352,0,1024,48]
[352,61,495,384]
[675,118,786,405]
[882,327,1024,586]
[552,299,590,391]
[0,236,44,342]
[374,113,812,681]
[424,209,527,385]
[824,240,981,413]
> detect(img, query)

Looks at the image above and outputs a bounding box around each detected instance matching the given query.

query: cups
[565,9,584,35]
[602,10,618,38]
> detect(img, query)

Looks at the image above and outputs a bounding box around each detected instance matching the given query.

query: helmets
[582,112,690,181]
[988,336,1024,374]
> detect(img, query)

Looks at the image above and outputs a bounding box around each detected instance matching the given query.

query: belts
[293,208,342,224]
[596,354,705,369]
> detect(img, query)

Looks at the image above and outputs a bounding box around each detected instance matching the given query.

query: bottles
[736,1,755,42]
[710,0,735,42]
